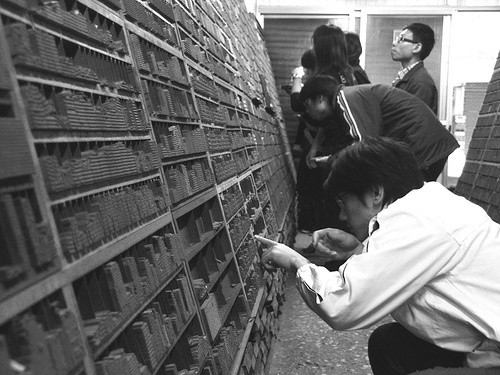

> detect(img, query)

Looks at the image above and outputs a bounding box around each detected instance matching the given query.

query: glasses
[336,191,352,204]
[395,35,419,44]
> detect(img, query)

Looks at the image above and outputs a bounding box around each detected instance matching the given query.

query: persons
[290,22,461,255]
[255,138,499,375]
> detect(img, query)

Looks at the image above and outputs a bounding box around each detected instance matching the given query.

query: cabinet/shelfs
[0,0,300,375]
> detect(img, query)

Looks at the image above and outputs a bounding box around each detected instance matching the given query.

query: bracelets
[293,74,302,80]
